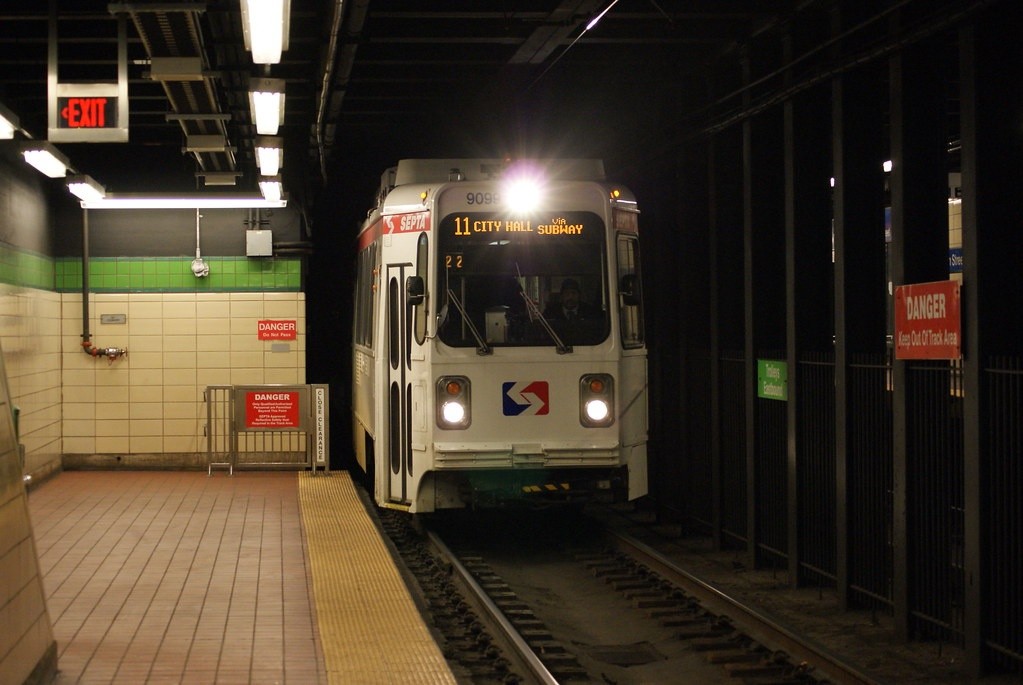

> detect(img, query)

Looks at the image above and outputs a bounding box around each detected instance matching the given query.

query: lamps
[239,0,291,64]
[78,191,288,208]
[257,172,284,200]
[0,103,22,142]
[66,173,106,202]
[254,136,284,177]
[19,141,71,178]
[247,76,287,135]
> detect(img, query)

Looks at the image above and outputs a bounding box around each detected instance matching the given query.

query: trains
[352,156,651,519]
